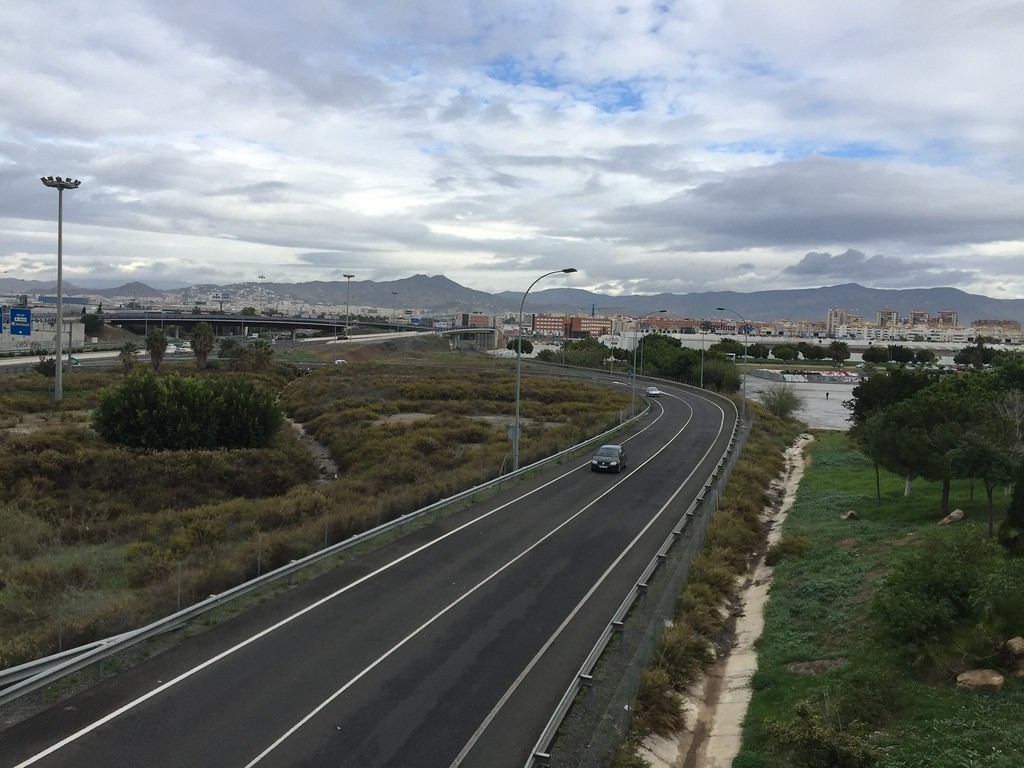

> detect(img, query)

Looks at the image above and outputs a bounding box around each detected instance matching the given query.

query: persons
[826,392,829,400]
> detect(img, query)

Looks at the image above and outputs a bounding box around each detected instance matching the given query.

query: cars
[590,444,628,473]
[646,386,660,397]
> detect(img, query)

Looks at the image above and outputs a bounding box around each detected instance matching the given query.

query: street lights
[717,307,748,416]
[512,267,577,471]
[700,325,715,388]
[41,174,83,405]
[630,309,667,422]
